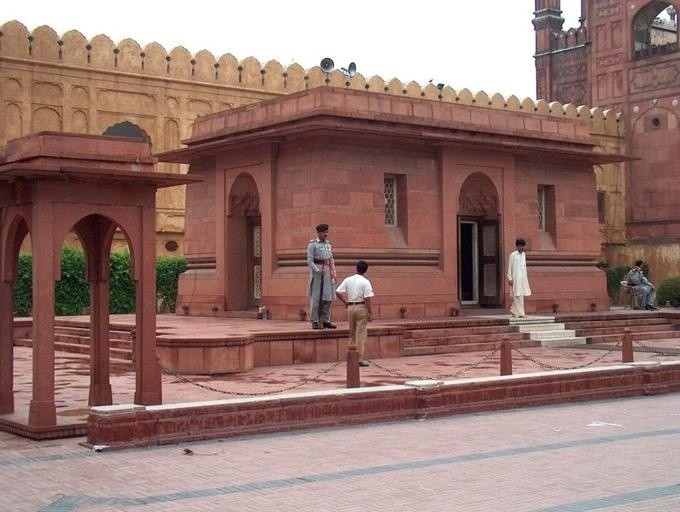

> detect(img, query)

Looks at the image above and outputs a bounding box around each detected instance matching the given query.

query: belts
[314,259,330,265]
[348,302,364,305]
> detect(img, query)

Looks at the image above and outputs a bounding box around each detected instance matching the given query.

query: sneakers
[642,303,656,310]
[312,322,318,329]
[323,322,336,328]
[359,361,369,366]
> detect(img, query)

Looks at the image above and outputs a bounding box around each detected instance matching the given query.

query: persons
[503,238,532,319]
[334,261,374,369]
[306,224,337,331]
[625,259,661,310]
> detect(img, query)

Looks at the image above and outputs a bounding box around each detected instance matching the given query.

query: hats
[635,259,643,266]
[316,224,328,232]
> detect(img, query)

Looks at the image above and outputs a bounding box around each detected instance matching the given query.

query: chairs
[620,281,642,310]
